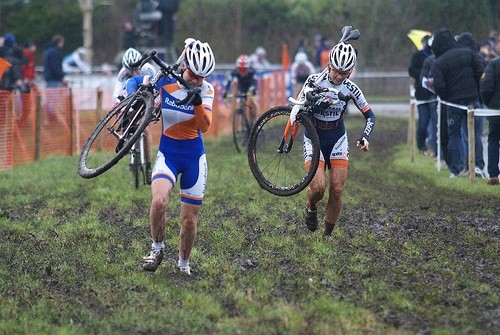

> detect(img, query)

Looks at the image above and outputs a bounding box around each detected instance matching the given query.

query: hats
[422,35,432,44]
[427,35,434,47]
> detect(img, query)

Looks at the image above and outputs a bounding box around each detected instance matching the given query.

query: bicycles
[223,91,267,155]
[75,49,202,179]
[246,86,349,196]
[115,94,150,189]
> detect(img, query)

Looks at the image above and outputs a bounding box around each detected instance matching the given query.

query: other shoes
[145,169,152,185]
[424,149,500,185]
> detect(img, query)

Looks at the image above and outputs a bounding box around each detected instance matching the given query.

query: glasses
[186,67,204,79]
[329,63,352,76]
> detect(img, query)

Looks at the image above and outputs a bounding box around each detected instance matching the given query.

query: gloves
[113,102,122,114]
[186,91,202,106]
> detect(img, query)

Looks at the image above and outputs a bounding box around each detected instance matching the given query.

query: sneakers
[142,248,164,272]
[306,207,318,232]
[179,266,190,274]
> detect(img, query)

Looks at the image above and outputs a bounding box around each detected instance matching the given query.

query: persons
[409,31,500,185]
[112,46,161,184]
[250,46,269,71]
[221,54,259,122]
[122,22,137,51]
[156,0,179,47]
[303,42,375,242]
[293,40,310,61]
[126,40,215,276]
[316,37,327,66]
[44,34,65,121]
[0,33,36,126]
[63,47,91,75]
[291,51,315,99]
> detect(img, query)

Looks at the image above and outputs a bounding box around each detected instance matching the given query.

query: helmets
[329,41,356,71]
[121,47,144,70]
[256,47,266,56]
[236,55,251,72]
[295,52,308,65]
[185,40,215,77]
